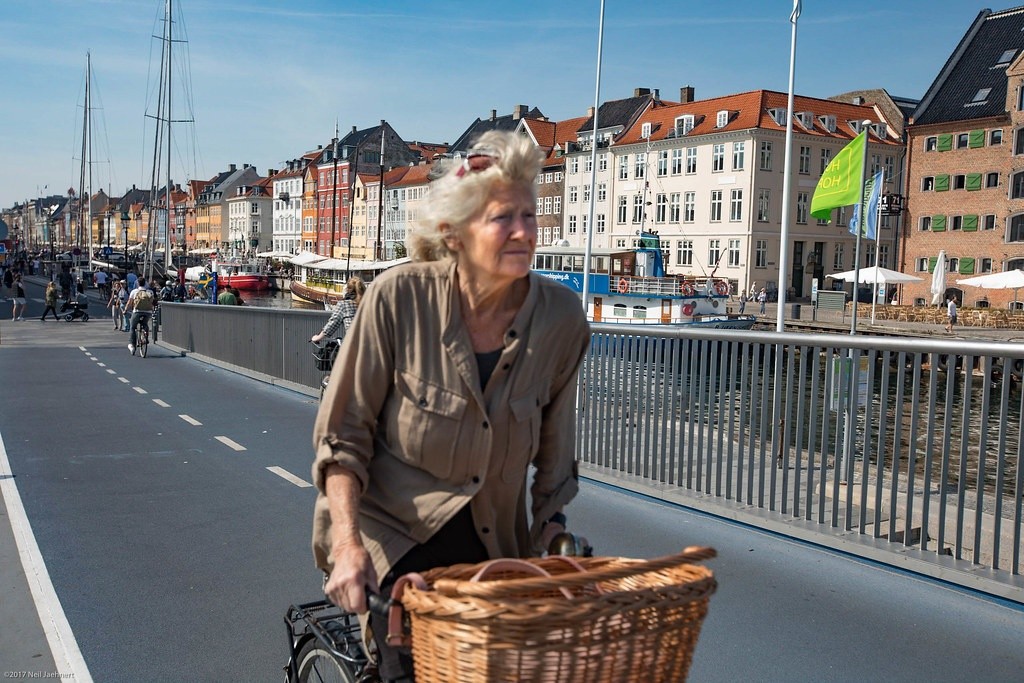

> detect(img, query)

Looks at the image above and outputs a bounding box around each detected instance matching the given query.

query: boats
[165,253,292,292]
[289,249,414,307]
[529,239,755,359]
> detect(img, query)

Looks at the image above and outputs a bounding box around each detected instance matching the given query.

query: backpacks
[162,287,173,301]
[134,288,152,311]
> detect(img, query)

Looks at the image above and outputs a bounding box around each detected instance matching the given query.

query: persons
[748,282,756,302]
[312,276,365,341]
[727,281,734,301]
[945,295,957,333]
[0,247,295,357]
[739,288,746,313]
[758,287,768,316]
[312,129,590,682]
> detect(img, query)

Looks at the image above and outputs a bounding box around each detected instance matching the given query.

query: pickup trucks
[56,251,86,261]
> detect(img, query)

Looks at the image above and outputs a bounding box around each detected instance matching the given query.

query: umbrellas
[956,269,1024,315]
[826,265,924,284]
[932,249,945,309]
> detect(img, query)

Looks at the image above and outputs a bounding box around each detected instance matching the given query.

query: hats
[120,280,126,284]
[166,281,172,285]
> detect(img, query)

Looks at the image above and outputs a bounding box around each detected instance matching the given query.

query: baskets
[402,545,718,683]
[311,338,339,372]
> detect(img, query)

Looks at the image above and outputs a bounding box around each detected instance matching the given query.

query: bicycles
[308,336,342,409]
[280,528,721,683]
[123,309,149,359]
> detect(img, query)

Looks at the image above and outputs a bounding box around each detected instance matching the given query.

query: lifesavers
[717,281,728,295]
[211,252,217,258]
[681,281,694,296]
[617,279,628,293]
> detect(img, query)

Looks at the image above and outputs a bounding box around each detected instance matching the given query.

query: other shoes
[13,318,18,321]
[18,317,25,321]
[113,326,129,333]
[57,318,60,321]
[41,318,45,322]
[128,343,135,353]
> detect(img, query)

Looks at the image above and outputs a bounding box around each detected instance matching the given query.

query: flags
[849,171,882,242]
[809,129,868,222]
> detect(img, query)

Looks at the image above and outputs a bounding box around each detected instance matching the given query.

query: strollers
[60,292,90,323]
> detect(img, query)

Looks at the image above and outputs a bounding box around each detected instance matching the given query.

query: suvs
[100,251,125,261]
